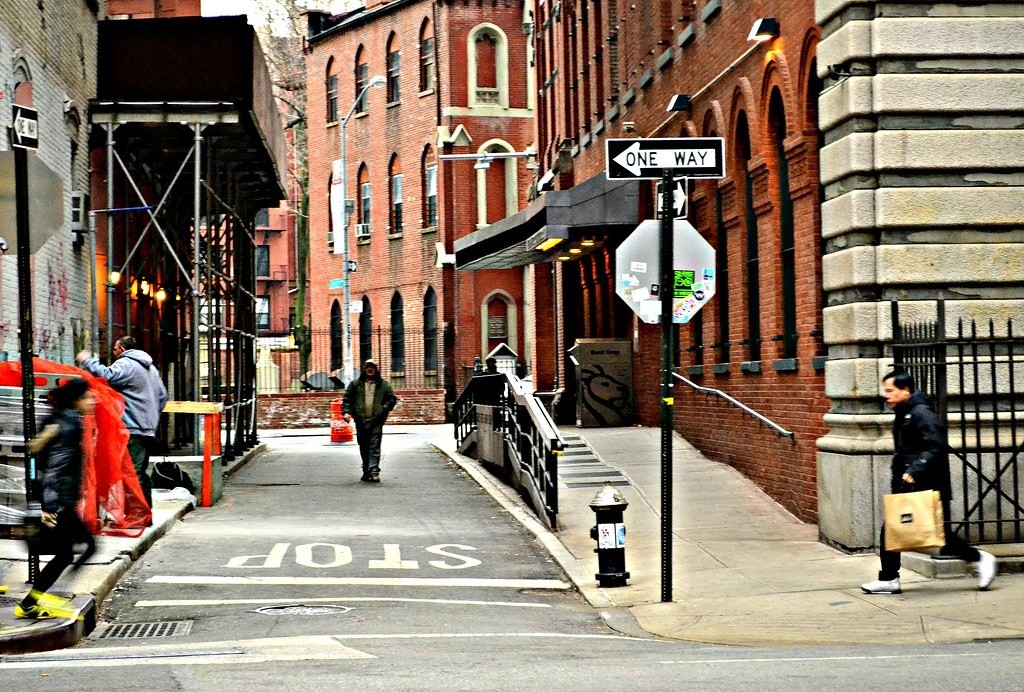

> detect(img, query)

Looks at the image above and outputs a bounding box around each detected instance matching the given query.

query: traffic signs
[653,178,689,219]
[606,137,726,180]
[5,104,41,151]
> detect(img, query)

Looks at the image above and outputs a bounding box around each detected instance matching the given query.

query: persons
[74,335,169,527]
[861,368,995,596]
[341,359,397,482]
[10,378,96,620]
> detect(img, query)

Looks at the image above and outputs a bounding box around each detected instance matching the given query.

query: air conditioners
[327,232,333,243]
[354,224,371,238]
[72,190,91,230]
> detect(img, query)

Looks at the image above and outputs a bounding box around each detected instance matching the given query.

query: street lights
[341,74,388,393]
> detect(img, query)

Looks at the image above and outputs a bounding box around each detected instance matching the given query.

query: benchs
[536,387,574,425]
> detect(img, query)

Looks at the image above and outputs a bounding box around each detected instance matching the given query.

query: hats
[364,359,377,367]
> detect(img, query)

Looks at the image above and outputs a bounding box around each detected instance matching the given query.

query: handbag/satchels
[883,488,947,552]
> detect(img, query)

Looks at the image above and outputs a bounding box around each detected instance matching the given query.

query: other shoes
[361,472,372,481]
[110,513,152,528]
[371,473,380,482]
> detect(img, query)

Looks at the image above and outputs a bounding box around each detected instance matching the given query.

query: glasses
[112,346,123,355]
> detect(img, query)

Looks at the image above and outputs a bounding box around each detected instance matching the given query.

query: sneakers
[861,577,902,594]
[13,604,56,620]
[974,549,998,589]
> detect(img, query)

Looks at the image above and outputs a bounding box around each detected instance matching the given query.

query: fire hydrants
[589,481,630,588]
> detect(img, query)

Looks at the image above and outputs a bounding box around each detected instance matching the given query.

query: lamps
[746,17,780,43]
[541,183,554,191]
[621,122,637,133]
[666,94,691,112]
